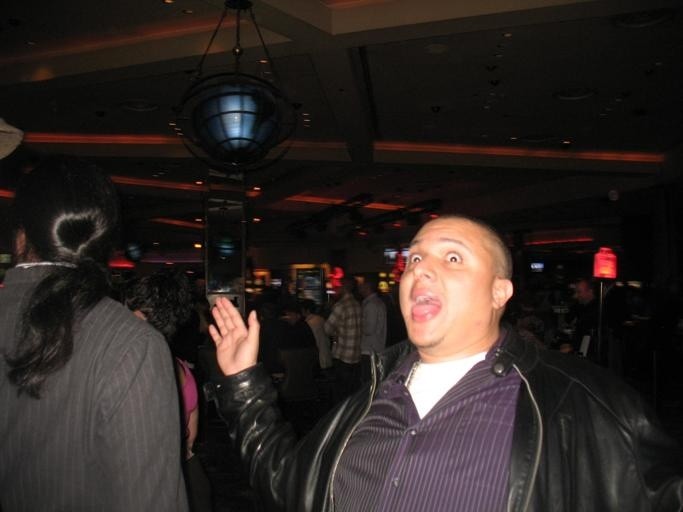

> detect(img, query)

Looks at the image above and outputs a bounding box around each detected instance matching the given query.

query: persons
[208,211,682,512]
[323,276,363,384]
[0,151,191,512]
[299,297,332,369]
[359,272,389,383]
[116,268,200,463]
[558,278,607,375]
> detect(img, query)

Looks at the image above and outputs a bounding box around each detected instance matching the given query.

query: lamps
[167,7,303,176]
